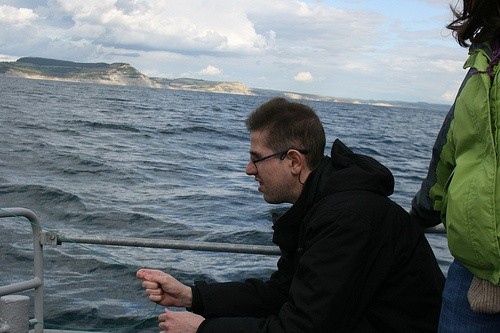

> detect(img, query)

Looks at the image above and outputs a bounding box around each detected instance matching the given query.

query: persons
[409,1,500,333]
[136,97,449,332]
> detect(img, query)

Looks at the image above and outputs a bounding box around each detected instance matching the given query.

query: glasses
[250,149,310,168]
